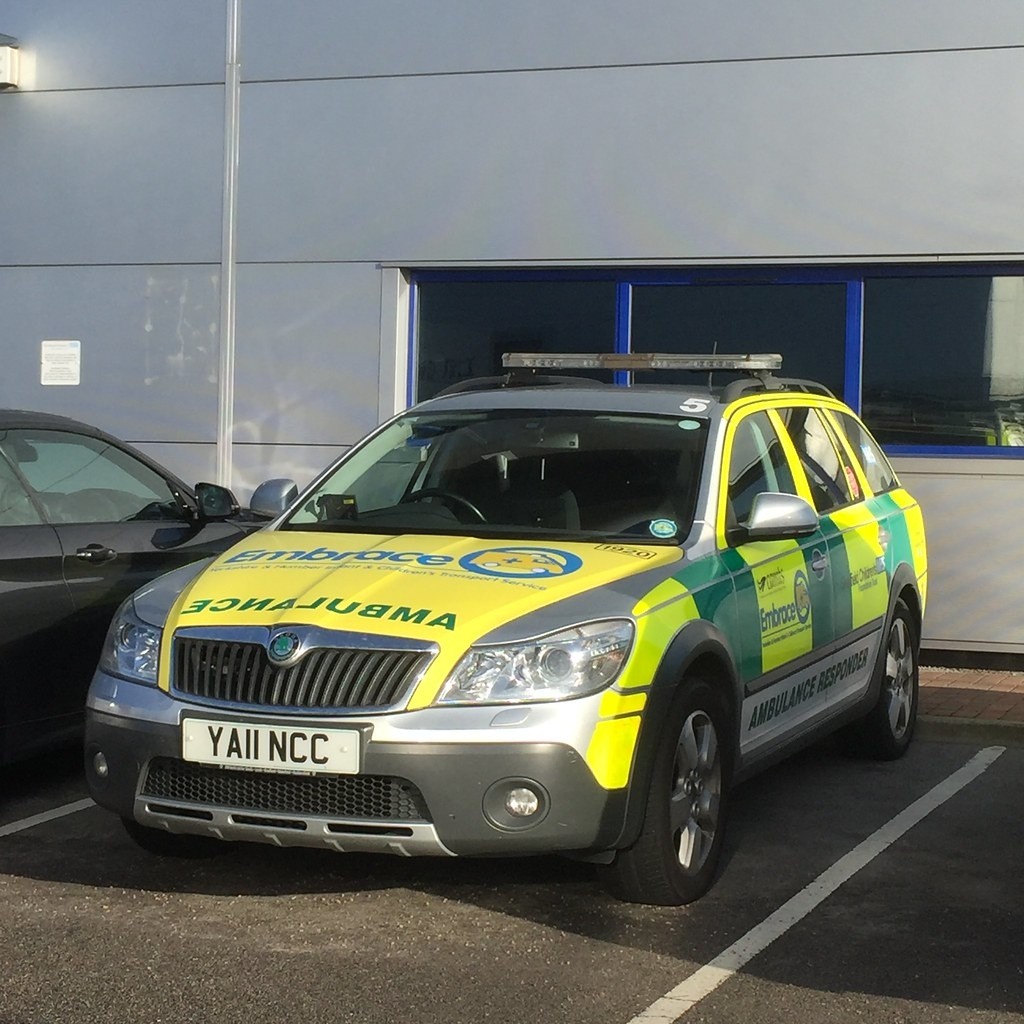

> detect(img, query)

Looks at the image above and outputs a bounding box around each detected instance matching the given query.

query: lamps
[0,34,20,89]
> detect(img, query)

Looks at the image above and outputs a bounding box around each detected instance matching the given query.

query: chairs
[462,445,580,531]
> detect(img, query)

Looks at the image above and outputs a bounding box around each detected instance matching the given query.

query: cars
[0,408,279,787]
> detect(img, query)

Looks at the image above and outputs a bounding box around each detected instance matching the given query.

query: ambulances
[82,352,931,905]
